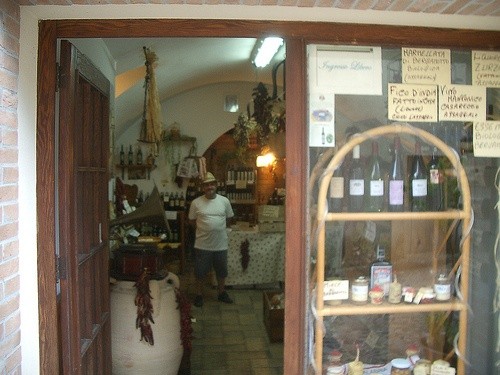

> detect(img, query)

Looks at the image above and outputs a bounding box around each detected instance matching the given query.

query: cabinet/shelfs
[135,209,194,276]
[115,163,157,180]
[310,120,474,375]
[186,158,260,223]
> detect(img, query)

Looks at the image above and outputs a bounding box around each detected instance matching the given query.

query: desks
[212,228,286,289]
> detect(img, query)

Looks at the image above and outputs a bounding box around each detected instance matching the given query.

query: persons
[187,172,238,303]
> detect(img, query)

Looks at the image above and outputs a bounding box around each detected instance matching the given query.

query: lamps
[250,37,284,70]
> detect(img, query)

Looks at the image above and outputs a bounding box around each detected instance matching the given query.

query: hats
[199,171,217,184]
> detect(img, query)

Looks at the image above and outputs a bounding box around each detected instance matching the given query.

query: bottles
[120,141,155,166]
[187,162,257,203]
[369,241,393,297]
[409,141,429,211]
[326,142,346,213]
[387,136,406,211]
[446,152,464,209]
[427,148,444,210]
[132,186,185,241]
[349,144,365,211]
[366,142,386,212]
[432,271,451,302]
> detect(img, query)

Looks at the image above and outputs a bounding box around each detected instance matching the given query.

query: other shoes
[217,292,233,304]
[195,295,204,307]
